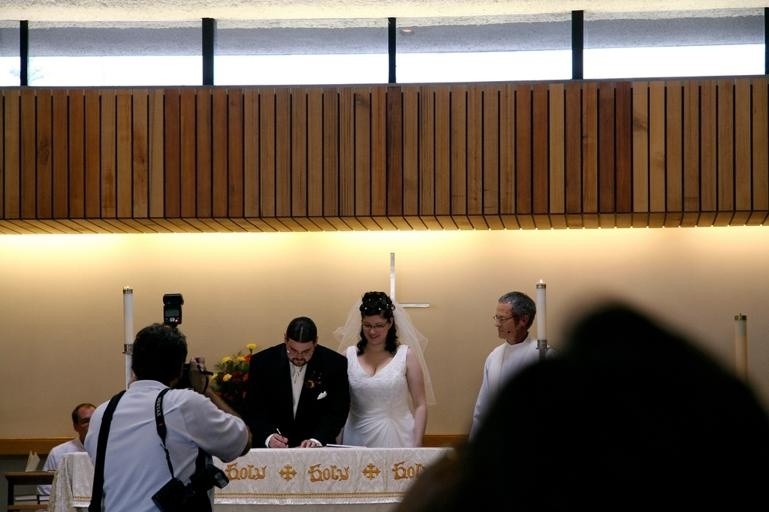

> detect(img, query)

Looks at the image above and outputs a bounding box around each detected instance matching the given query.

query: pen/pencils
[276,428,289,448]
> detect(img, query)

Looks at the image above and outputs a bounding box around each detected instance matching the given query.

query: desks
[3,470,55,511]
[64,448,457,512]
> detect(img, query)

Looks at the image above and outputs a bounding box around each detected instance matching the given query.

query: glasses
[363,319,387,329]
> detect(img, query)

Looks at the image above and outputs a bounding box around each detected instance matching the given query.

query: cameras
[161,292,201,391]
[151,463,229,512]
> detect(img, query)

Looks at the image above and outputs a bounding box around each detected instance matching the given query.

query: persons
[332,291,437,448]
[36,403,97,503]
[83,323,253,512]
[466,290,560,449]
[244,317,351,448]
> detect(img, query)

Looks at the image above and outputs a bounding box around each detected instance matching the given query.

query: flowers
[210,342,258,400]
